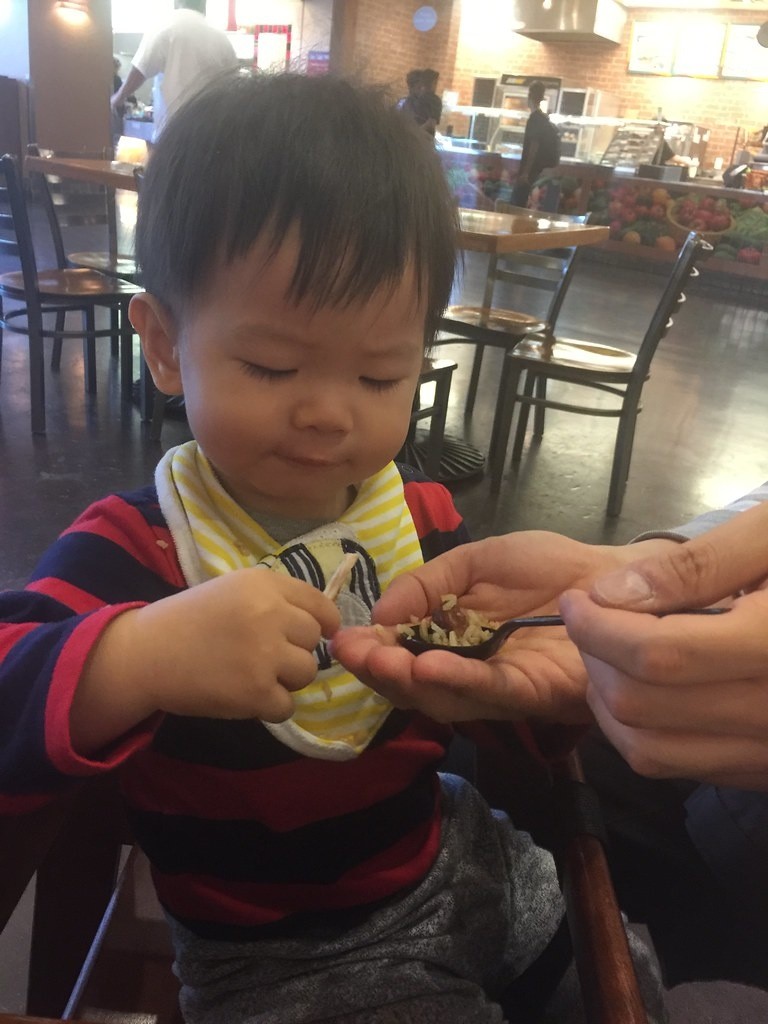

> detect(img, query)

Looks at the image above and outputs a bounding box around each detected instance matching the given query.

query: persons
[2,68,676,1024]
[507,81,557,210]
[111,57,138,146]
[392,70,445,142]
[327,475,767,990]
[111,0,240,145]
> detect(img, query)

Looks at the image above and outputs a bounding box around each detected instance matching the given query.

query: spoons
[398,606,732,662]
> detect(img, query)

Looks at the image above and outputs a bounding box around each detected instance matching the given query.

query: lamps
[55,0,89,24]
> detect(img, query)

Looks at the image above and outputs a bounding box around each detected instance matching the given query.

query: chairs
[26,142,149,400]
[432,208,592,456]
[2,723,645,1024]
[0,151,149,435]
[487,230,716,514]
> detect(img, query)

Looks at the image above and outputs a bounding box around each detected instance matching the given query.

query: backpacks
[537,120,562,168]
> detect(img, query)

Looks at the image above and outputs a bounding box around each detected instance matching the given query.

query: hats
[528,80,549,101]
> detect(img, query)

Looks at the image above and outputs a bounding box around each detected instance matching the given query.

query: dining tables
[22,154,610,487]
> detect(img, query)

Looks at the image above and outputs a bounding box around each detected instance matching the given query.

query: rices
[397,594,500,647]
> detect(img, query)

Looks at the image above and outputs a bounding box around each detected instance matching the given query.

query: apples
[605,183,678,251]
[679,194,732,230]
[738,245,763,267]
